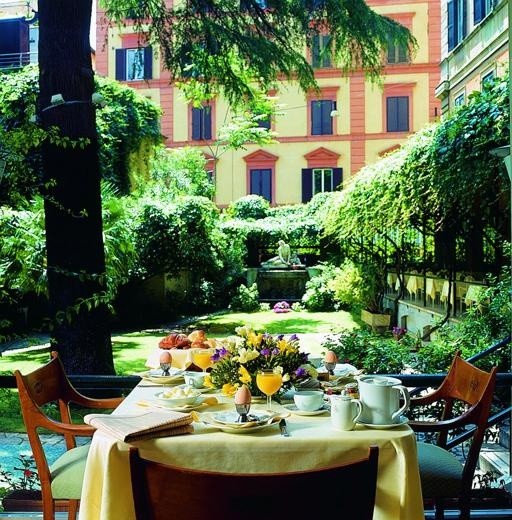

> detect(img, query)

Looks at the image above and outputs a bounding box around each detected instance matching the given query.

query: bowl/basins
[155,388,201,406]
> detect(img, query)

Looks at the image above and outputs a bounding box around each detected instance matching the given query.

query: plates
[180,383,212,395]
[209,410,269,434]
[284,404,328,417]
[356,413,410,429]
[161,398,208,412]
[143,372,181,385]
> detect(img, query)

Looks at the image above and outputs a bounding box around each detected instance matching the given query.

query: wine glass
[255,368,283,417]
[192,348,216,373]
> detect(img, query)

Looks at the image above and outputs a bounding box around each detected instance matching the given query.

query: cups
[356,374,411,424]
[182,371,211,387]
[291,390,326,412]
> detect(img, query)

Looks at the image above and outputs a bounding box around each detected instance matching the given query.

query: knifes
[279,418,289,436]
[136,383,177,387]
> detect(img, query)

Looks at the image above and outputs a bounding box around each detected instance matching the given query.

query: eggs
[325,351,336,362]
[160,352,172,363]
[235,387,251,404]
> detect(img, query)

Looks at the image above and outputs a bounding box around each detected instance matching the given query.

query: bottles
[331,388,363,433]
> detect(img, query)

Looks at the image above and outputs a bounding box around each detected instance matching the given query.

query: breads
[159,330,209,349]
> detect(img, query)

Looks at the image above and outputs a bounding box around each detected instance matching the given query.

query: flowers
[205,327,320,398]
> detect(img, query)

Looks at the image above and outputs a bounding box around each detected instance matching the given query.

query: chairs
[122,444,383,518]
[398,349,499,519]
[14,347,125,520]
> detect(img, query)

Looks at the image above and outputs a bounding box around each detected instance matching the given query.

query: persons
[260,239,292,266]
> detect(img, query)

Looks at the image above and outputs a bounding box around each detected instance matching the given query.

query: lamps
[329,102,341,118]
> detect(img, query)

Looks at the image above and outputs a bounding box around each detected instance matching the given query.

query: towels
[82,407,194,444]
